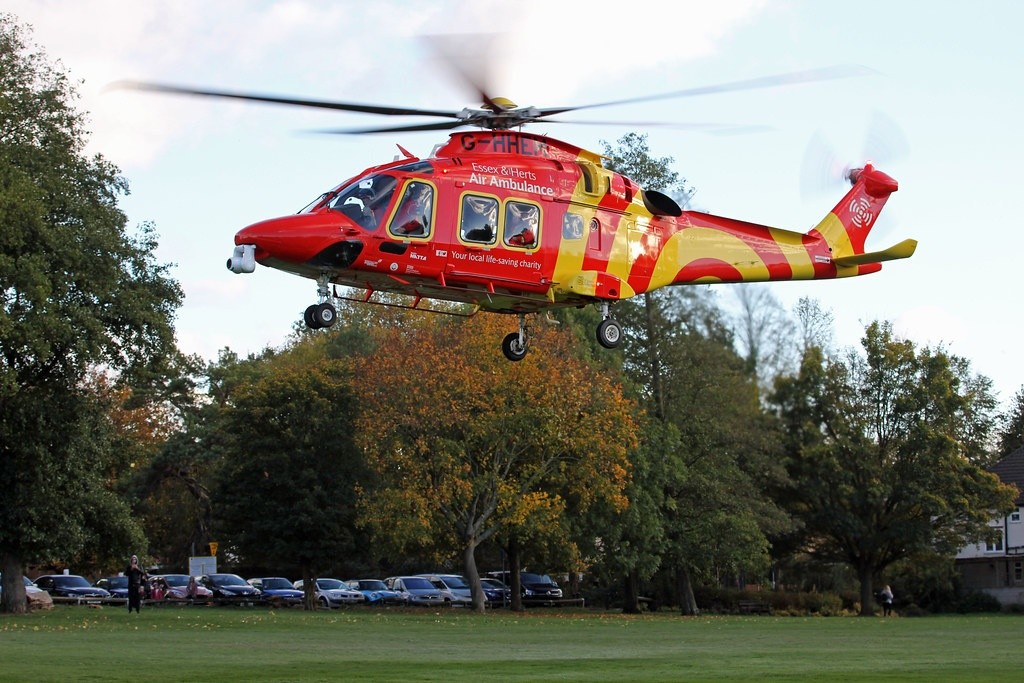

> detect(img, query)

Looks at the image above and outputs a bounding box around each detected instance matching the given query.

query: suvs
[487,570,563,605]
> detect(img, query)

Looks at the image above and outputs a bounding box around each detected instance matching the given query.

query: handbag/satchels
[881,594,887,601]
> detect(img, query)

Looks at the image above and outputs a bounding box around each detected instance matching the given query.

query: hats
[160,584,164,587]
[153,583,158,586]
[131,555,137,564]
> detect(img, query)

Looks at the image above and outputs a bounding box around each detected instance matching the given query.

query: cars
[382,576,444,608]
[293,577,366,608]
[0,574,42,602]
[30,575,113,606]
[337,578,403,607]
[148,574,214,607]
[415,574,489,607]
[193,572,262,607]
[88,576,134,606]
[246,577,305,605]
[479,577,512,608]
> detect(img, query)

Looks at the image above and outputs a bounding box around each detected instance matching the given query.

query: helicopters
[100,32,922,362]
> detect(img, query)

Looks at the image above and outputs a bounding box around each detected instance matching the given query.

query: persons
[360,183,421,235]
[124,555,199,613]
[881,584,894,616]
[503,212,535,247]
